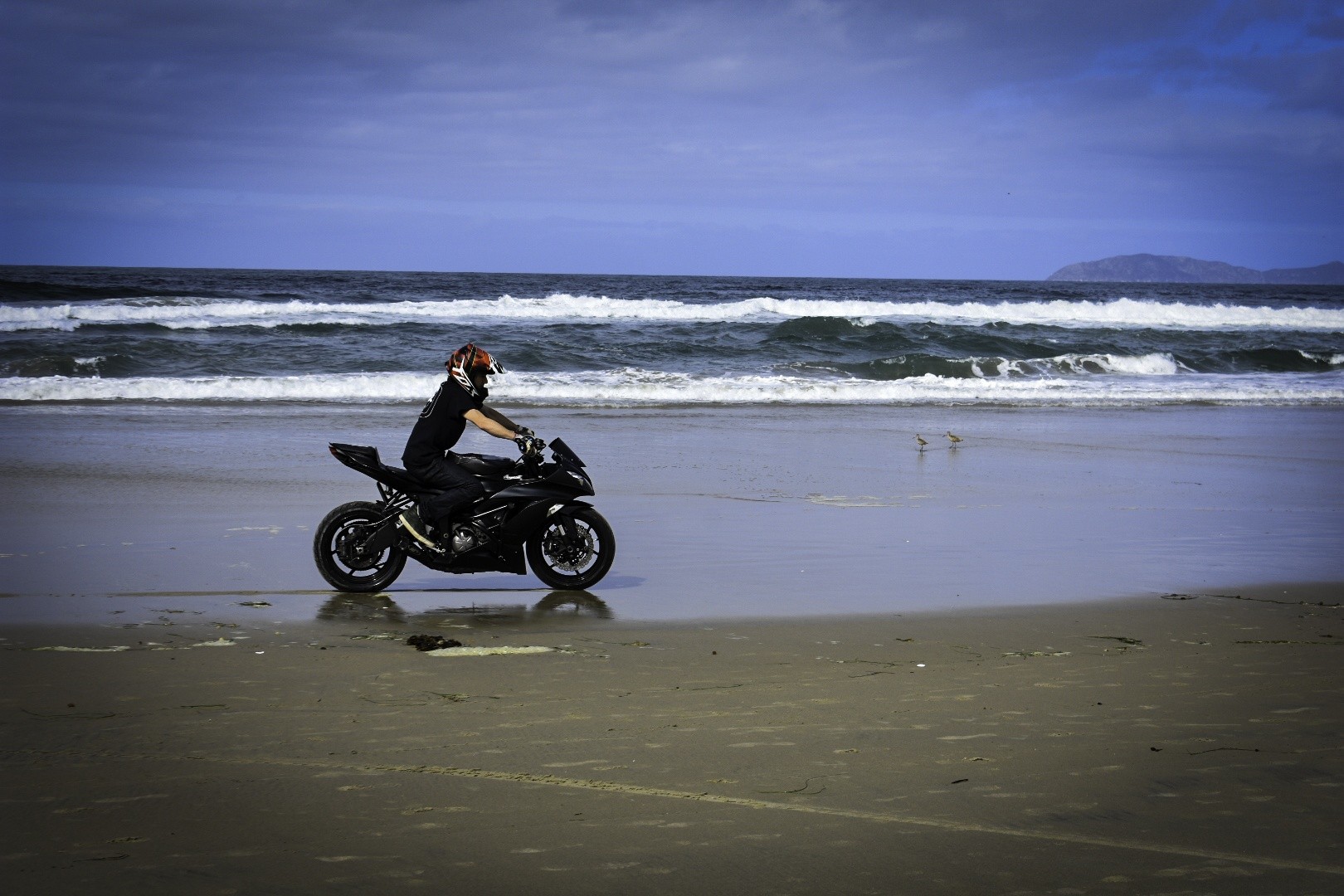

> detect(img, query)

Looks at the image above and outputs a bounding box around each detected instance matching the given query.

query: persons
[398,345,537,547]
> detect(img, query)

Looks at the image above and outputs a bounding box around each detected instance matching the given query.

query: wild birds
[942,431,963,449]
[913,433,929,452]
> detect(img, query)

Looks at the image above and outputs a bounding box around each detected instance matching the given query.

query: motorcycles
[313,434,616,593]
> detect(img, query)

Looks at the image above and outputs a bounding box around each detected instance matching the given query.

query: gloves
[515,424,535,436]
[514,434,537,458]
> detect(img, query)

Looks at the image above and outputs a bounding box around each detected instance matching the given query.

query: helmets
[445,343,507,402]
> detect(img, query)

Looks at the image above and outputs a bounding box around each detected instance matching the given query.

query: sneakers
[399,503,436,546]
